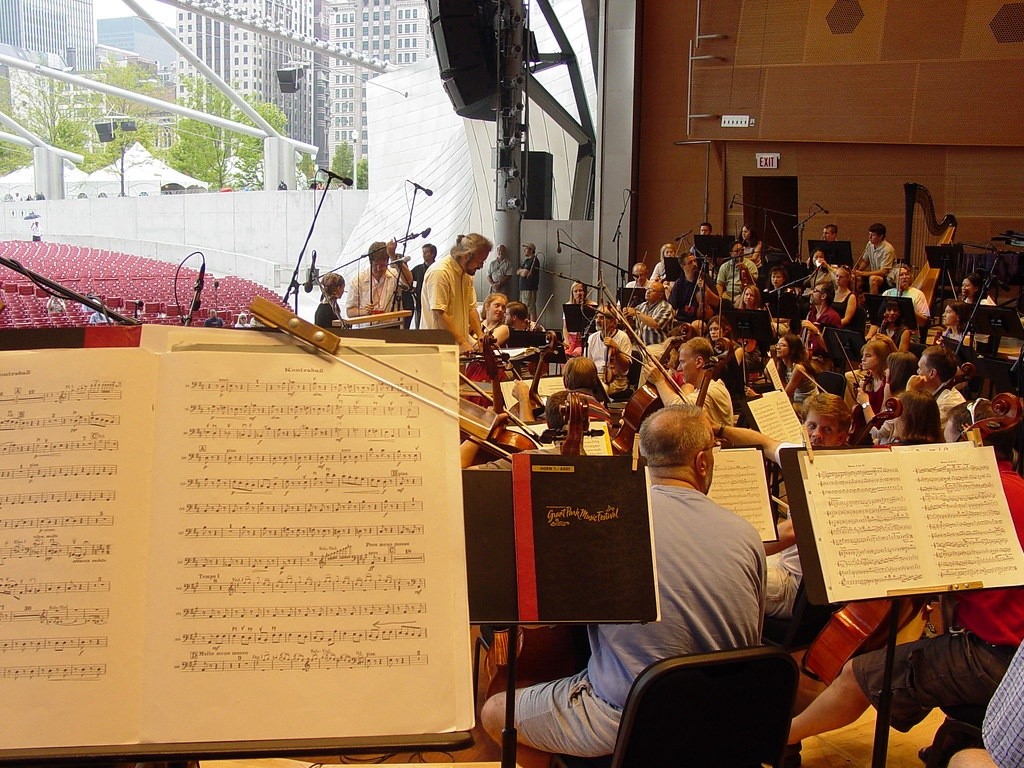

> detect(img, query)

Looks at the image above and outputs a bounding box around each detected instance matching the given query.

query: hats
[521,243,537,250]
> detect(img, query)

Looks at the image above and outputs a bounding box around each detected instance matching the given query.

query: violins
[846,369,874,446]
[572,293,589,350]
[624,295,638,338]
[802,305,816,361]
[738,247,758,291]
[695,270,714,320]
[879,313,889,335]
[736,302,770,354]
[850,272,865,307]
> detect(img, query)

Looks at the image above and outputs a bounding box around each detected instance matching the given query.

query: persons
[30,220,43,241]
[204,309,266,327]
[309,182,324,189]
[461,221,1024,614]
[80,291,112,322]
[158,302,167,318]
[481,405,769,768]
[779,400,1024,768]
[14,192,45,201]
[314,232,493,354]
[46,291,67,314]
[278,180,287,190]
[135,300,147,315]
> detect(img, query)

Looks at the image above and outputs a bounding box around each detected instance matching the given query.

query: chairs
[550,646,799,768]
[760,576,846,654]
[757,252,788,280]
[908,343,927,359]
[0,240,294,329]
[609,350,643,402]
[814,370,846,399]
[850,307,866,334]
[926,715,982,768]
[861,259,907,292]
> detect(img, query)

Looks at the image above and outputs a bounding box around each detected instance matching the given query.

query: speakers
[520,151,553,220]
[277,67,304,92]
[95,121,118,142]
[428,0,499,121]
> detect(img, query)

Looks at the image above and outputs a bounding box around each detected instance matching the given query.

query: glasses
[647,287,662,293]
[731,247,744,253]
[775,343,788,347]
[841,265,850,270]
[813,288,823,294]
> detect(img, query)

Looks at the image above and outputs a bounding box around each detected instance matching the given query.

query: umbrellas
[309,178,324,182]
[23,212,41,220]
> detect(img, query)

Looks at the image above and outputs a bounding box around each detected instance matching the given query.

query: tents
[86,141,208,198]
[1,159,90,199]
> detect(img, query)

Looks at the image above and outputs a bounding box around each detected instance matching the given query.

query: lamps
[506,12,522,211]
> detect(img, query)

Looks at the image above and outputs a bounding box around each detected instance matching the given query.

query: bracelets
[717,422,725,437]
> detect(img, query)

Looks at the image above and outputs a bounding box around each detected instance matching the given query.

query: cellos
[957,391,1024,442]
[508,329,559,425]
[946,362,976,391]
[478,335,538,460]
[611,322,696,457]
[852,394,904,447]
[696,336,735,408]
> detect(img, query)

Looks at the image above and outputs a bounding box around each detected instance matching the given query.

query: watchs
[862,402,869,409]
[636,310,640,316]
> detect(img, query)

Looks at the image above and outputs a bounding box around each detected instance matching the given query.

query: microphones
[413,183,433,196]
[556,230,561,253]
[527,256,537,280]
[305,252,316,293]
[729,196,735,209]
[627,188,639,198]
[991,246,1000,256]
[422,228,431,238]
[388,256,411,265]
[675,230,691,241]
[322,169,353,186]
[583,304,612,319]
[190,263,205,310]
[803,267,820,286]
[815,203,829,214]
